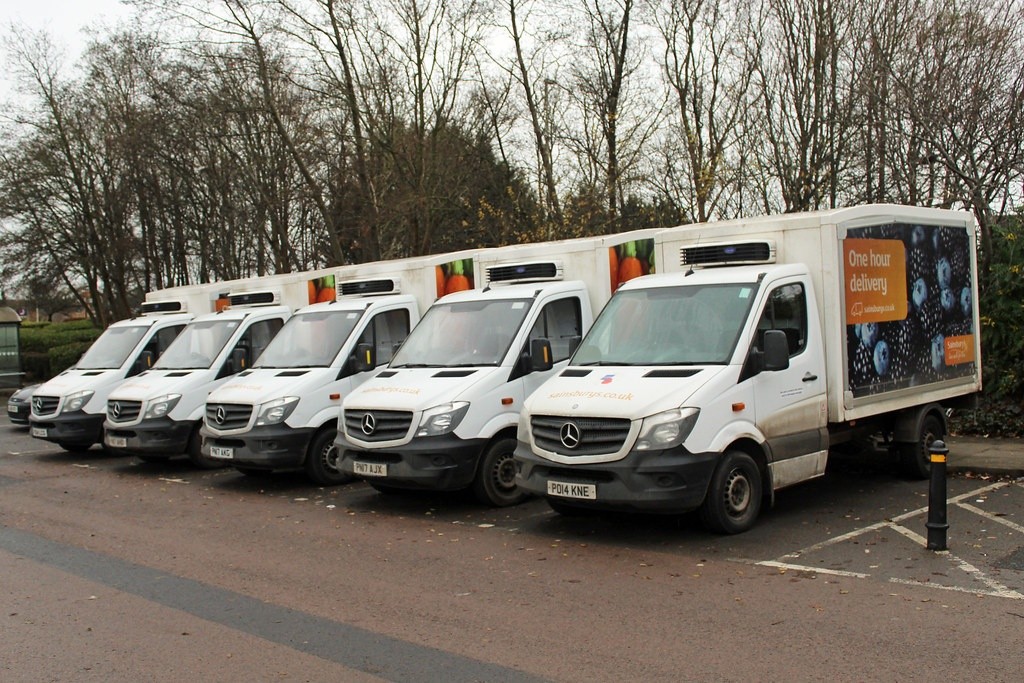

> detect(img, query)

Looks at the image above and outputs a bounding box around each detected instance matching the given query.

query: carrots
[609,237,657,294]
[436,258,475,300]
[308,275,338,305]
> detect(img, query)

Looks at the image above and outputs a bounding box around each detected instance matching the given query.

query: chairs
[758,300,799,355]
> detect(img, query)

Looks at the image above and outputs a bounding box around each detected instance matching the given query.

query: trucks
[512,201,984,536]
[101,263,350,471]
[333,227,668,505]
[199,248,494,484]
[27,279,235,456]
